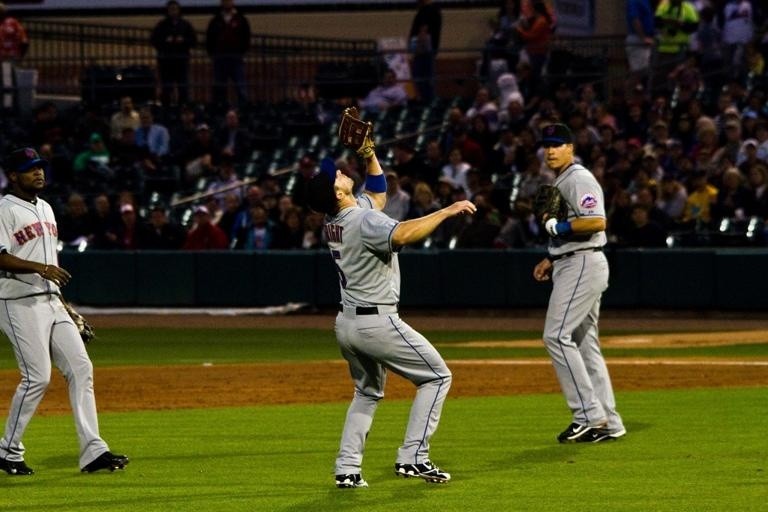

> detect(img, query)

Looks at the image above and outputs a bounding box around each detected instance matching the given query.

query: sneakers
[557,422,618,445]
[395,460,453,483]
[80,452,129,473]
[0,458,35,477]
[334,474,370,490]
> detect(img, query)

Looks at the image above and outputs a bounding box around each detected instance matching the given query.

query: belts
[548,247,602,262]
[336,302,379,316]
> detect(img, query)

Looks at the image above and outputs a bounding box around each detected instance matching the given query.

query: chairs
[0,73,768,256]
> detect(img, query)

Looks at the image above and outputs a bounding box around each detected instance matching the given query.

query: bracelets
[41,265,48,277]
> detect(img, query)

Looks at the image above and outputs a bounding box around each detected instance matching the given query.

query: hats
[306,157,338,214]
[1,147,51,176]
[536,122,575,146]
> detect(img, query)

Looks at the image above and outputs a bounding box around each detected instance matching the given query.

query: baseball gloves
[64,305,95,346]
[338,107,375,160]
[531,184,563,226]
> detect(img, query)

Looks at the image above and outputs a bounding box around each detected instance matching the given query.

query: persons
[0,147,129,475]
[307,107,479,488]
[533,124,626,444]
[1,0,767,250]
